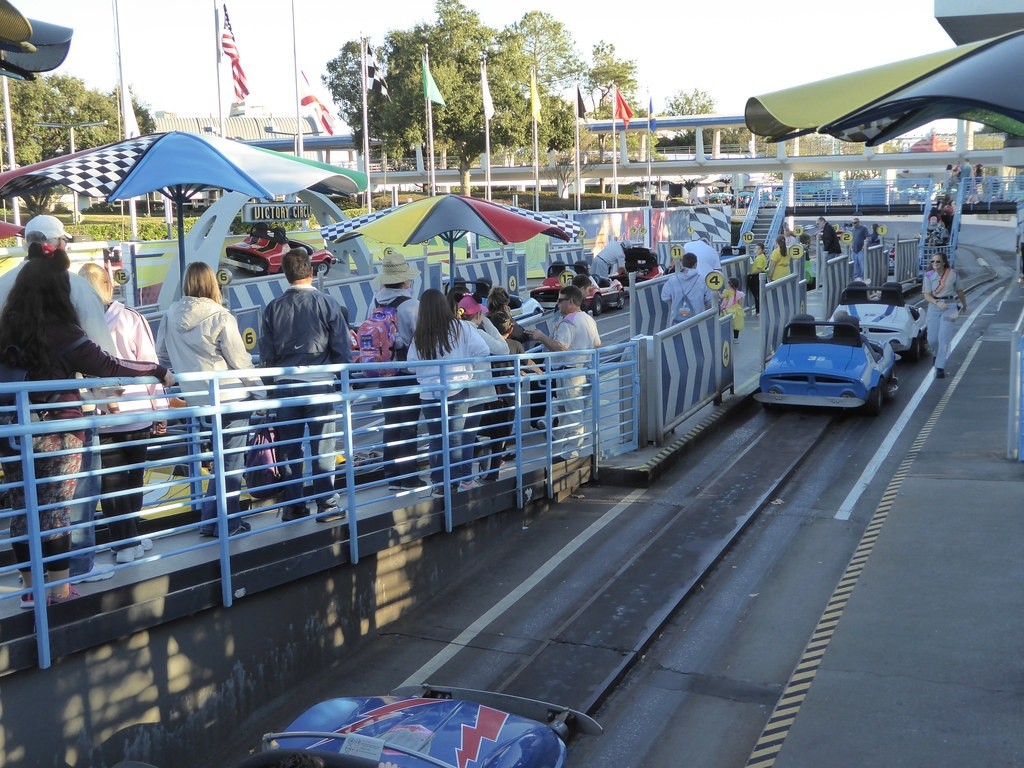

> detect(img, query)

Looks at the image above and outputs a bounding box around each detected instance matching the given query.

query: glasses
[558,298,569,303]
[852,222,858,224]
[816,222,821,225]
[931,260,943,263]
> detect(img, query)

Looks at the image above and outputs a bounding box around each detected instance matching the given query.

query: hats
[24,215,73,242]
[372,253,421,285]
[454,292,489,315]
[621,240,631,249]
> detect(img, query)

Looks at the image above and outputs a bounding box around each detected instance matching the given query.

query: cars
[238,680,604,768]
[752,282,930,415]
[223,222,342,278]
[530,241,670,318]
[441,279,546,331]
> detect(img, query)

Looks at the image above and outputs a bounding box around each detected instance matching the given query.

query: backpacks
[357,296,412,378]
[672,274,700,325]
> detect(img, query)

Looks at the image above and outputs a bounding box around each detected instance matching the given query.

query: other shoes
[315,503,346,522]
[111,538,153,562]
[732,338,739,344]
[199,520,251,537]
[432,484,458,498]
[19,585,35,608]
[458,479,484,493]
[69,562,115,585]
[501,448,516,461]
[281,505,310,521]
[932,357,945,377]
[536,420,557,441]
[388,475,427,491]
[47,583,80,606]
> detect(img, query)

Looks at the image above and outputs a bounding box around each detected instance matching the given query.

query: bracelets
[935,301,939,305]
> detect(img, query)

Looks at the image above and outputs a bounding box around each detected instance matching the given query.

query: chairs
[881,281,904,307]
[256,222,267,235]
[845,281,868,299]
[274,227,288,244]
[833,316,859,344]
[789,314,818,337]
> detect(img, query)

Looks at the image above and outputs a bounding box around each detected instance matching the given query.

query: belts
[556,366,575,370]
[597,255,607,263]
[934,296,953,299]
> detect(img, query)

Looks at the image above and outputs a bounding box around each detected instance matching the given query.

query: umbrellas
[5,130,368,305]
[319,193,581,289]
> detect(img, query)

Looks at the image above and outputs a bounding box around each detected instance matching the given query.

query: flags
[577,86,587,120]
[649,95,657,133]
[531,74,543,124]
[220,3,249,103]
[299,56,336,135]
[614,89,633,130]
[481,67,495,121]
[364,42,392,102]
[421,57,446,107]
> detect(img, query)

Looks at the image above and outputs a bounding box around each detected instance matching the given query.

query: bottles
[394,333,403,349]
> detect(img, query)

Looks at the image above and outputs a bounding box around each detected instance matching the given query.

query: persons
[0,209,882,608]
[921,252,968,379]
[924,157,983,254]
